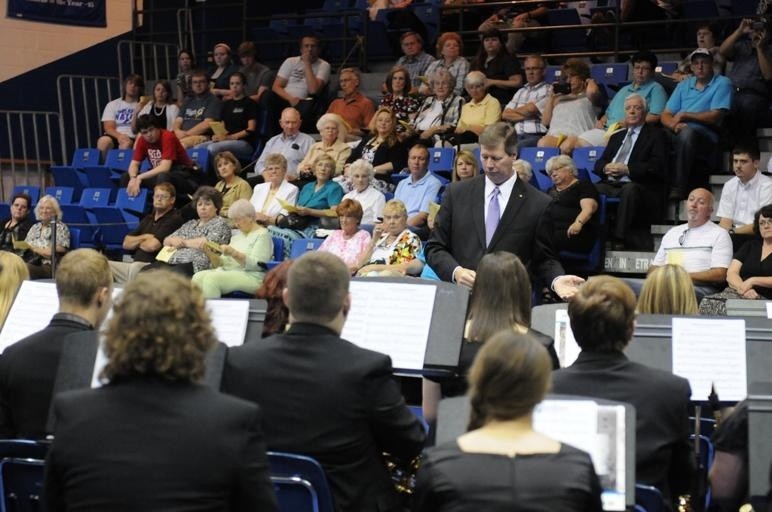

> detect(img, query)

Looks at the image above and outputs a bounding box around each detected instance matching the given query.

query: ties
[486,187,499,249]
[616,130,633,163]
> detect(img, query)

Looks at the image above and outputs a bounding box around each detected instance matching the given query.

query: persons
[0,247,119,439]
[419,250,562,429]
[401,327,604,511]
[705,397,751,511]
[38,267,282,511]
[5,0,772,340]
[546,272,706,512]
[220,252,430,511]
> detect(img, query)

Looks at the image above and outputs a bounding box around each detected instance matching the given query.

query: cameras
[553,81,571,94]
[750,21,767,30]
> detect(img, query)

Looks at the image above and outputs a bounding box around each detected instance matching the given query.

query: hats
[691,48,712,61]
